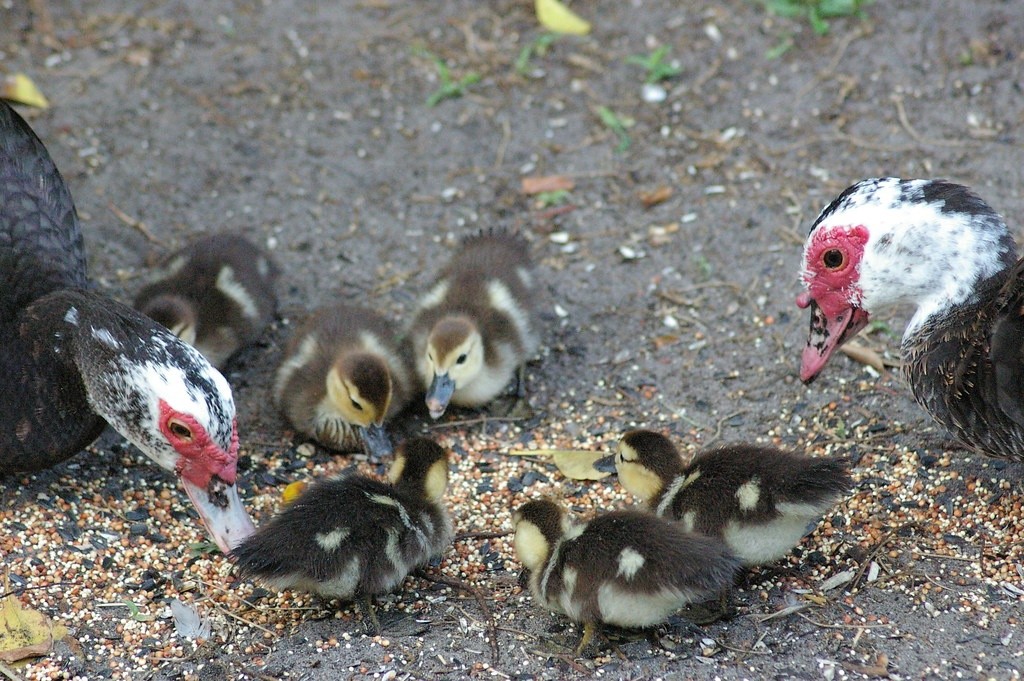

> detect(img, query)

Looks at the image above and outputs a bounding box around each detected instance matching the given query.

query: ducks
[0,96,553,641]
[794,175,1024,464]
[510,427,860,662]
